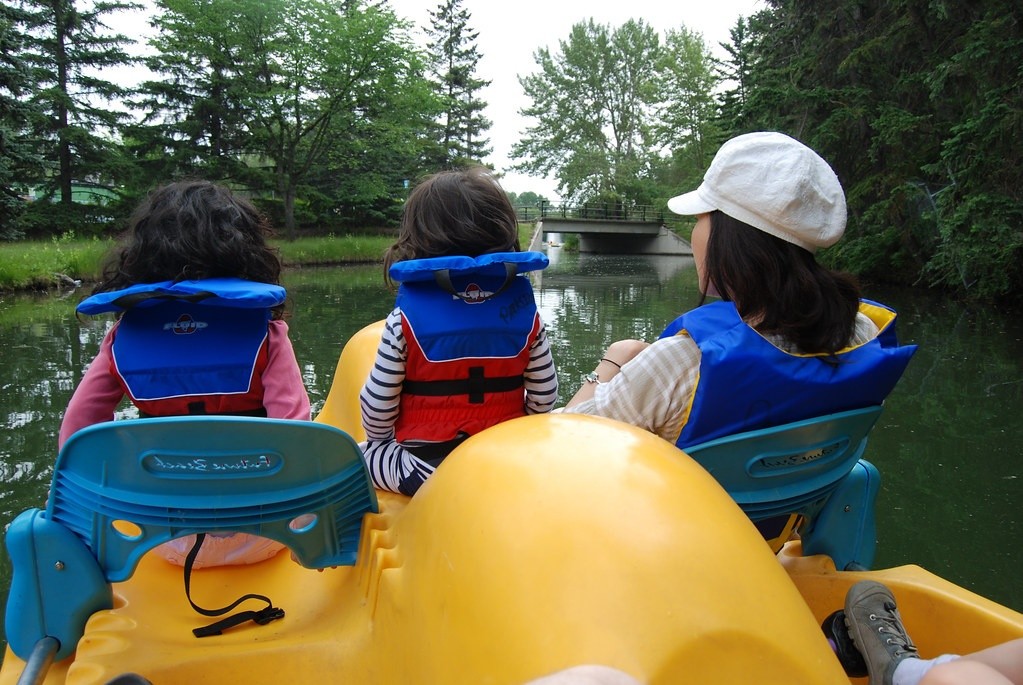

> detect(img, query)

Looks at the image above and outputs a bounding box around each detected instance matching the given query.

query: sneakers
[844,580,920,685]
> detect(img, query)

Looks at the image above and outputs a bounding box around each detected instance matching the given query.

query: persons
[355,166,559,497]
[844,579,1023,685]
[557,130,916,554]
[57,180,338,573]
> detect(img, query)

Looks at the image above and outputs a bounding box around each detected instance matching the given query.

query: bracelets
[587,371,601,384]
[601,358,621,369]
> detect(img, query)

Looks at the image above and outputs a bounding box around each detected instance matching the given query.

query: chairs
[678,399,888,557]
[4,416,381,659]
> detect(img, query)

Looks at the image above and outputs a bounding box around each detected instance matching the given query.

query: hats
[667,132,847,255]
